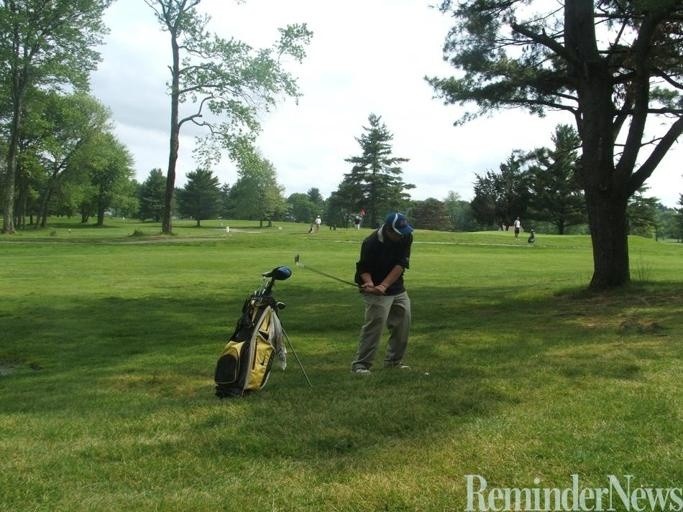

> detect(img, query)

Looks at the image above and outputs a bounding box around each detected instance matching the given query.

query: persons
[513,216,520,239]
[314,215,321,231]
[350,211,413,375]
[354,215,360,230]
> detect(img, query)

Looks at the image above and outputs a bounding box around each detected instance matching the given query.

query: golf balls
[424,371,429,378]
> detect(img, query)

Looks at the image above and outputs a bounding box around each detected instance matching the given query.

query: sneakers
[395,363,409,369]
[350,368,370,374]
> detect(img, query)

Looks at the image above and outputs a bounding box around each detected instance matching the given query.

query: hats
[386,212,412,236]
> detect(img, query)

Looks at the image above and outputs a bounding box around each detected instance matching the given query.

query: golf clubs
[294,254,369,289]
[249,265,292,321]
